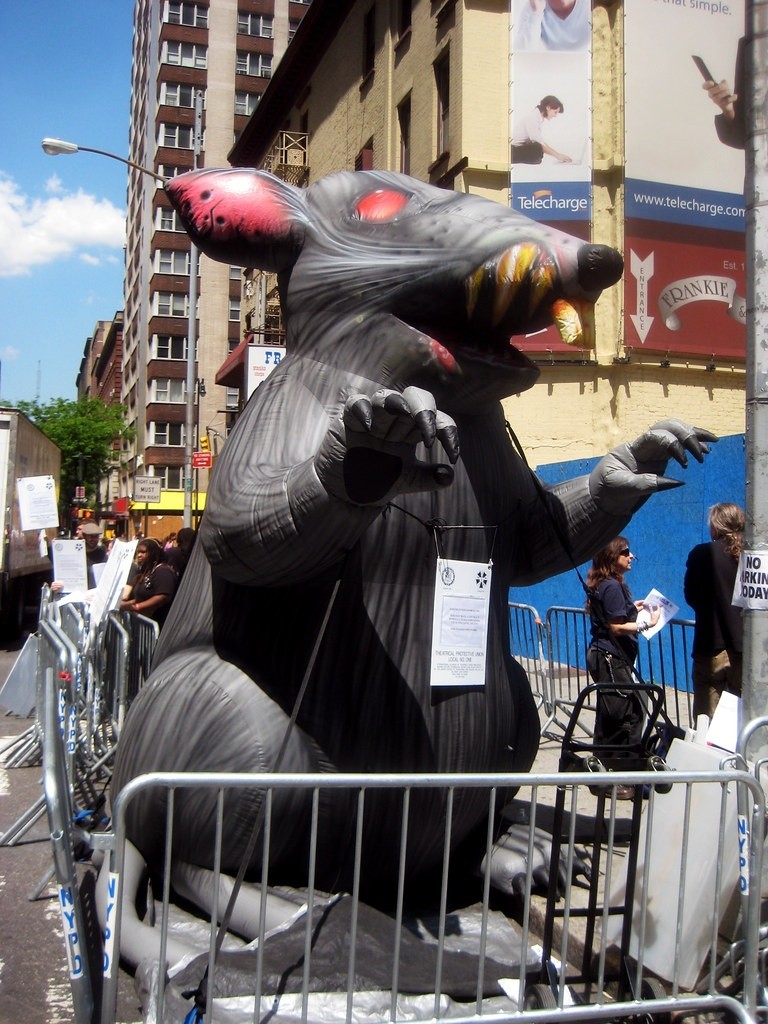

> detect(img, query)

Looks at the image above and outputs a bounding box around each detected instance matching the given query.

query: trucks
[0,406,62,649]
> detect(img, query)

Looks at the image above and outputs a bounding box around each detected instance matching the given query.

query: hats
[83,523,104,535]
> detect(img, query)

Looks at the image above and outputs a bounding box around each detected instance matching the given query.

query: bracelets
[636,621,649,633]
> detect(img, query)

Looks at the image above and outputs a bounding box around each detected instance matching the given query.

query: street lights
[40,138,198,531]
[193,376,206,530]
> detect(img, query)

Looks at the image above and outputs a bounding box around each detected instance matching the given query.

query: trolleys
[524,683,672,1023]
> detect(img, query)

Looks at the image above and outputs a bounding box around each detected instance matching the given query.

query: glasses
[618,548,630,557]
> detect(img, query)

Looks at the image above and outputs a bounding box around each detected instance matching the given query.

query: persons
[515,0,590,52]
[514,95,571,162]
[121,539,176,699]
[703,36,744,151]
[166,527,195,575]
[685,504,745,729]
[586,536,660,800]
[163,532,176,551]
[45,519,115,602]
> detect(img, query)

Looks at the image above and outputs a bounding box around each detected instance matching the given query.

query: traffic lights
[79,509,95,518]
[200,437,211,452]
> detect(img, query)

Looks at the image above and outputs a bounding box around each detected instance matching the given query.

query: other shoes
[606,785,635,800]
